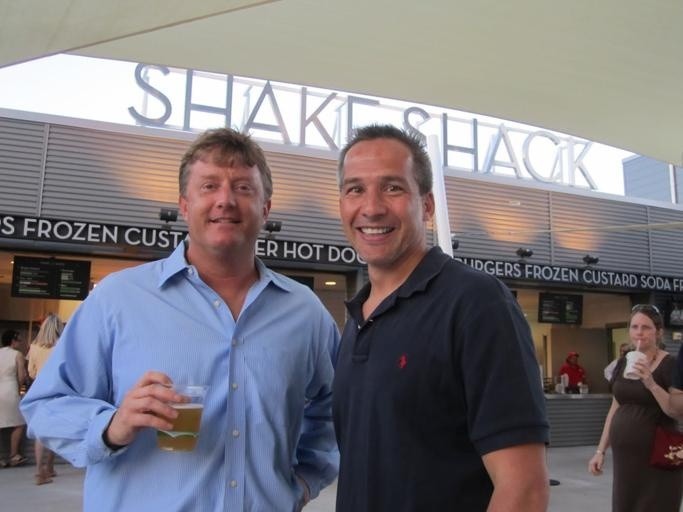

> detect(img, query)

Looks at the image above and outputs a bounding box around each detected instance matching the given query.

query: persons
[587,303,683,512]
[333,122,550,512]
[559,351,587,388]
[25,313,64,485]
[19,126,343,512]
[0,329,26,467]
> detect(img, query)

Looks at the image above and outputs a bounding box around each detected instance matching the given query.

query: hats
[567,351,580,359]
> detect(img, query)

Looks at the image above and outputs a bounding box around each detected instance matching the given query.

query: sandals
[0,454,30,467]
[35,470,57,486]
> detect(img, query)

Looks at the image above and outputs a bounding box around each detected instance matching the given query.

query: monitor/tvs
[538,291,584,324]
[286,275,317,291]
[10,255,91,300]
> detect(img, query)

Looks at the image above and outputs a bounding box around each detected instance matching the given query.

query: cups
[156,383,208,453]
[624,350,647,381]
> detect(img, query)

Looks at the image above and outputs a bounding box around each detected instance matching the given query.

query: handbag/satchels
[649,424,683,469]
